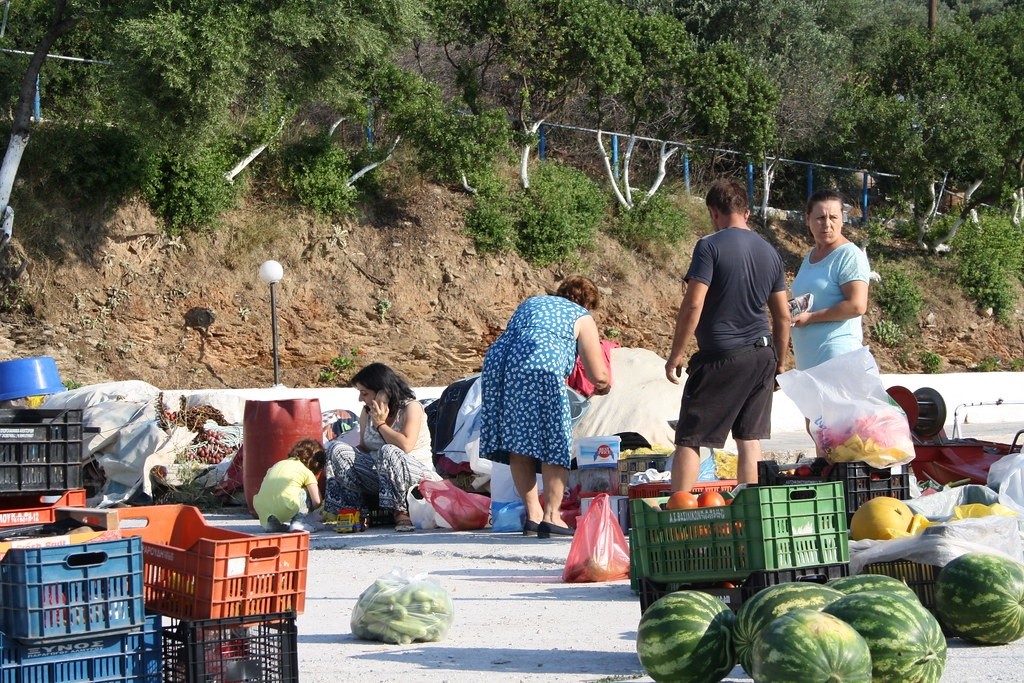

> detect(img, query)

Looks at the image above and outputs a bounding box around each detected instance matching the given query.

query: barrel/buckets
[242,398,325,517]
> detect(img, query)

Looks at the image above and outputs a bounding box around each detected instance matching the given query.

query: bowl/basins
[575,435,622,470]
[0,357,68,402]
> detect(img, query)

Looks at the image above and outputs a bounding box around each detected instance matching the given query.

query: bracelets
[377,422,386,430]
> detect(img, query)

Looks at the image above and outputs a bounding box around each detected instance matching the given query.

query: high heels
[523,519,539,535]
[537,521,575,538]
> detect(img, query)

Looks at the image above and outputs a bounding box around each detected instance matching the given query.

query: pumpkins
[849,495,914,540]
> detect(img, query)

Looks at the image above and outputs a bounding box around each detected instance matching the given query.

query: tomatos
[165,412,233,464]
[667,490,743,542]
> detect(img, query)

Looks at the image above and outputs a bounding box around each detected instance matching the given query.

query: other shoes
[393,510,413,532]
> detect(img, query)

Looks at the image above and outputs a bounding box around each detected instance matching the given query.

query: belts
[755,336,772,347]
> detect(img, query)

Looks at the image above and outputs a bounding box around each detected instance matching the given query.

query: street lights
[259,259,285,384]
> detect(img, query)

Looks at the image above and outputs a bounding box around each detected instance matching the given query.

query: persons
[326,363,435,531]
[665,176,791,496]
[481,274,609,537]
[791,188,870,459]
[254,439,326,533]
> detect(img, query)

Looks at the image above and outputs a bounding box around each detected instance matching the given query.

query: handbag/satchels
[786,292,814,327]
[406,458,571,532]
[350,572,453,644]
[563,493,630,581]
[776,344,916,469]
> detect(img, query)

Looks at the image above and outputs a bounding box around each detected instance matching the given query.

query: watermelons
[933,552,1023,644]
[635,574,948,683]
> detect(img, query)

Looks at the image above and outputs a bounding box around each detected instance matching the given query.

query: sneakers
[289,513,317,532]
[265,518,289,532]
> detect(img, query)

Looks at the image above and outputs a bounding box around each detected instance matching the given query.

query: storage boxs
[576,452,952,637]
[0,407,310,683]
